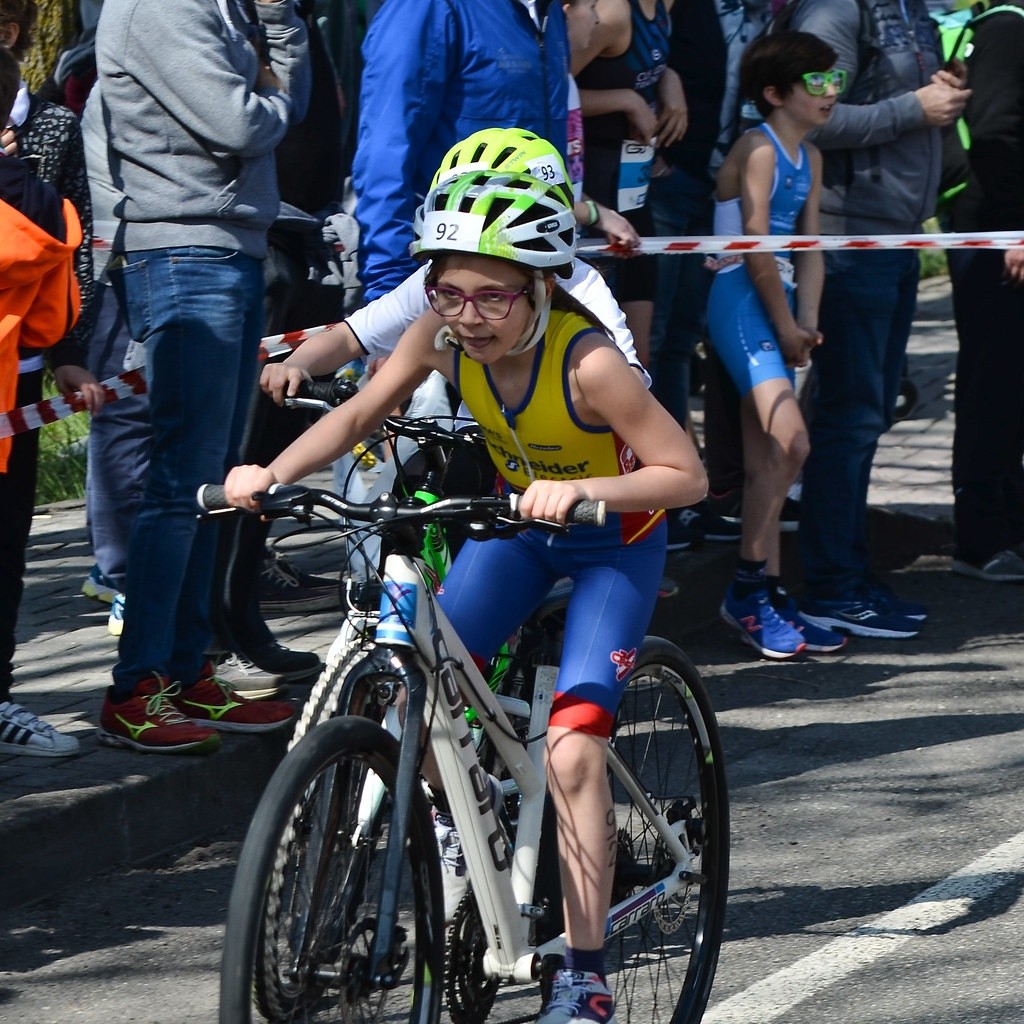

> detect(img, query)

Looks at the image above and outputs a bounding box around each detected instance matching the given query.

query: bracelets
[585,200,599,225]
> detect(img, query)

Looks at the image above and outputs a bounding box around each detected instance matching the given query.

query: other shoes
[667,528,689,552]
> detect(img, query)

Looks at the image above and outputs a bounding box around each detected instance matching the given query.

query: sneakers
[950,542,1023,579]
[109,598,130,635]
[673,498,747,540]
[257,557,341,612]
[81,563,122,602]
[171,663,293,731]
[430,772,503,923]
[769,587,849,651]
[535,968,617,1024]
[0,699,80,757]
[95,669,220,754]
[798,591,922,640]
[209,650,288,700]
[268,548,345,594]
[719,586,810,658]
[702,483,800,532]
[248,640,321,682]
[865,572,931,622]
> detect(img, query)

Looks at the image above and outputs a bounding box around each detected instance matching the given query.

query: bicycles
[193,474,729,1024]
[248,371,597,1024]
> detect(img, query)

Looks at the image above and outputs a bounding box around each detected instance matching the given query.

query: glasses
[423,282,535,320]
[801,68,847,97]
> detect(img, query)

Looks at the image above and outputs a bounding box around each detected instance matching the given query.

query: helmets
[411,168,577,279]
[426,129,573,211]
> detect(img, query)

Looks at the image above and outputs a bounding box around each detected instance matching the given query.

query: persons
[0,0,1024,758]
[255,127,655,800]
[222,172,710,1024]
